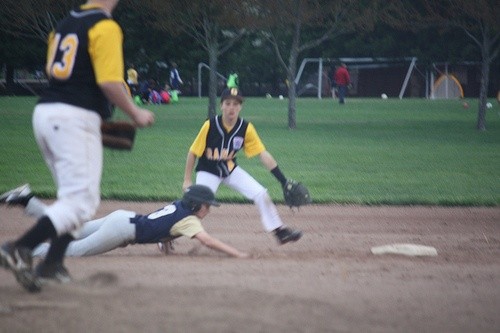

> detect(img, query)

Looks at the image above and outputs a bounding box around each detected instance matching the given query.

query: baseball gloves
[100,120,135,151]
[282,179,312,216]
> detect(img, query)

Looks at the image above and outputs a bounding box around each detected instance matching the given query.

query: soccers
[486,103,492,109]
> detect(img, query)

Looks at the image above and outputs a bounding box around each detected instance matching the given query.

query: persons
[134,84,180,103]
[332,65,350,104]
[0,186,254,258]
[127,65,139,93]
[223,72,241,95]
[157,87,310,250]
[169,65,183,94]
[0,0,155,292]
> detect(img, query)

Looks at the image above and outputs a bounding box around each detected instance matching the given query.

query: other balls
[463,103,469,109]
